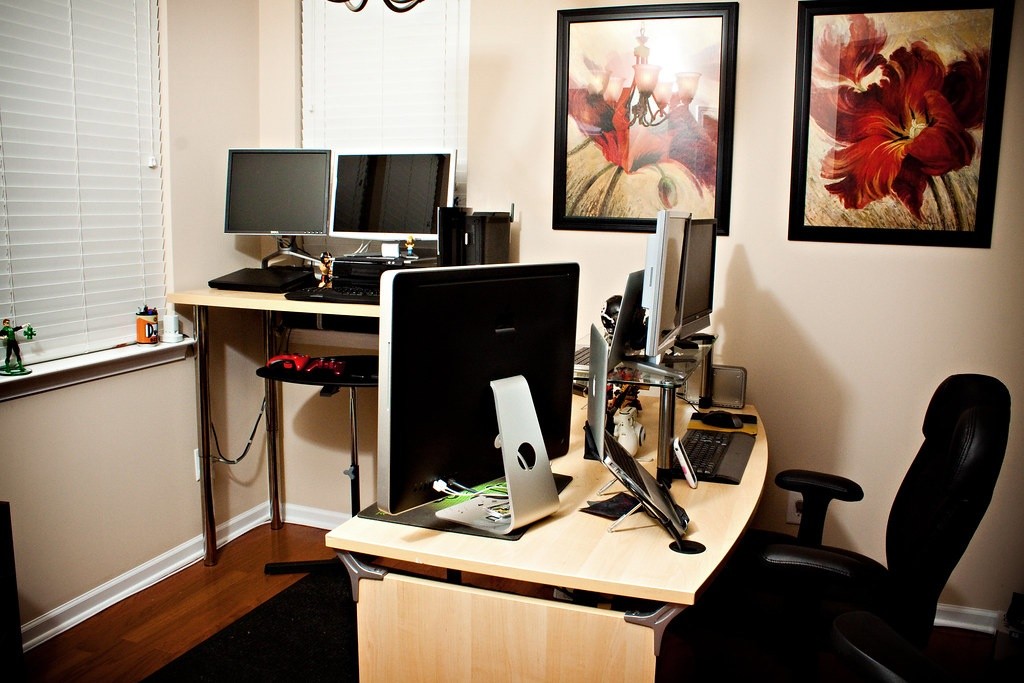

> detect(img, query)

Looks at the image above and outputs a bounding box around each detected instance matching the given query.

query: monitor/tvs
[623,210,719,379]
[223,147,457,269]
[378,261,581,535]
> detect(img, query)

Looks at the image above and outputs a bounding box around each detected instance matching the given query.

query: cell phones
[674,437,697,490]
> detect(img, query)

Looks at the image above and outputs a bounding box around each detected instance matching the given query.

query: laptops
[586,324,686,538]
[574,269,646,372]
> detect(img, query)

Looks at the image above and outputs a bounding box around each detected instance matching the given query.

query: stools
[257,353,379,578]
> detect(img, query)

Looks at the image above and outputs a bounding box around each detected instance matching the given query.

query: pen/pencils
[143,305,149,315]
[137,307,141,314]
[148,309,153,315]
[152,306,158,315]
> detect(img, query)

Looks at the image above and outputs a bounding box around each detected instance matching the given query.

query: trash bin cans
[991,592,1024,676]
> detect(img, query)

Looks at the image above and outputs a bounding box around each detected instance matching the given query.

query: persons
[0,319,30,371]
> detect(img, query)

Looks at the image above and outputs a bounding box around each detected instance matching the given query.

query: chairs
[669,373,1011,683]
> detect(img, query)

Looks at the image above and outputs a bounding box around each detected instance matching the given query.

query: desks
[171,287,380,567]
[324,378,769,683]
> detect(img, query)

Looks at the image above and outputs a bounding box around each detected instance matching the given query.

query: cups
[136,312,158,343]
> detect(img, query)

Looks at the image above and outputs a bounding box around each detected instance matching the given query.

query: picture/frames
[553,2,739,236]
[787,0,1015,248]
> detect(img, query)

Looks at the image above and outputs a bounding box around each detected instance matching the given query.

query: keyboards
[286,286,380,305]
[674,429,757,484]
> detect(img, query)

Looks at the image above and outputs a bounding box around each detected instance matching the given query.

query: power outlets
[785,490,803,525]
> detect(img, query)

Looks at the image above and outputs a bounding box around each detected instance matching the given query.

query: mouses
[703,409,744,429]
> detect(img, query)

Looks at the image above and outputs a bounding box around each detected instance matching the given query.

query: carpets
[139,563,526,683]
